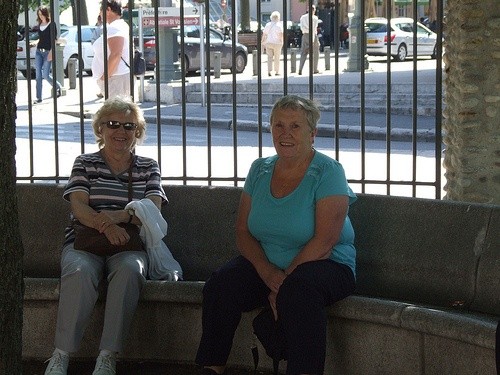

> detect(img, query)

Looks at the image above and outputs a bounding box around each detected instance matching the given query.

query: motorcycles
[285,18,328,52]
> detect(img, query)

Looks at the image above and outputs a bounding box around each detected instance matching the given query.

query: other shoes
[197,368,228,375]
[314,70,319,74]
[299,72,302,75]
[274,73,280,76]
[56,86,62,98]
[268,73,272,76]
[34,99,43,104]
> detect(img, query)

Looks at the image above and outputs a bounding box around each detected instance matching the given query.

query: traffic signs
[143,7,202,15]
[142,17,201,26]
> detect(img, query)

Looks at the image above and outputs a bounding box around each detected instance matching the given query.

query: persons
[298,5,321,75]
[262,11,283,76]
[33,5,63,103]
[90,0,135,103]
[42,95,182,375]
[195,95,357,375]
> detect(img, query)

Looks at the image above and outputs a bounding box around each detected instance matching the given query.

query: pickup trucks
[235,32,263,54]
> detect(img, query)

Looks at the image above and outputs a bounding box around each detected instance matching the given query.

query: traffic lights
[221,0,228,8]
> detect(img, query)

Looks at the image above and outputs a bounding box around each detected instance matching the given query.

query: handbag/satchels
[73,219,142,256]
[133,49,147,78]
[251,308,287,375]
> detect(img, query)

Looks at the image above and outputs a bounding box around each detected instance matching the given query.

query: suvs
[15,23,75,78]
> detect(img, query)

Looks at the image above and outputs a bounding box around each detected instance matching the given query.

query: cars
[364,17,445,61]
[144,24,249,79]
[48,25,102,76]
[418,16,445,32]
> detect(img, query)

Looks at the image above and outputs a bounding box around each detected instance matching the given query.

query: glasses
[102,120,137,131]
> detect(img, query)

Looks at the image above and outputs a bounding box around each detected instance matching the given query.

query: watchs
[126,207,134,225]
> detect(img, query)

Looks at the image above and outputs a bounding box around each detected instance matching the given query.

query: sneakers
[93,351,117,375]
[43,352,70,375]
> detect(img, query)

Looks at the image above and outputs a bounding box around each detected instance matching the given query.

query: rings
[102,221,104,224]
